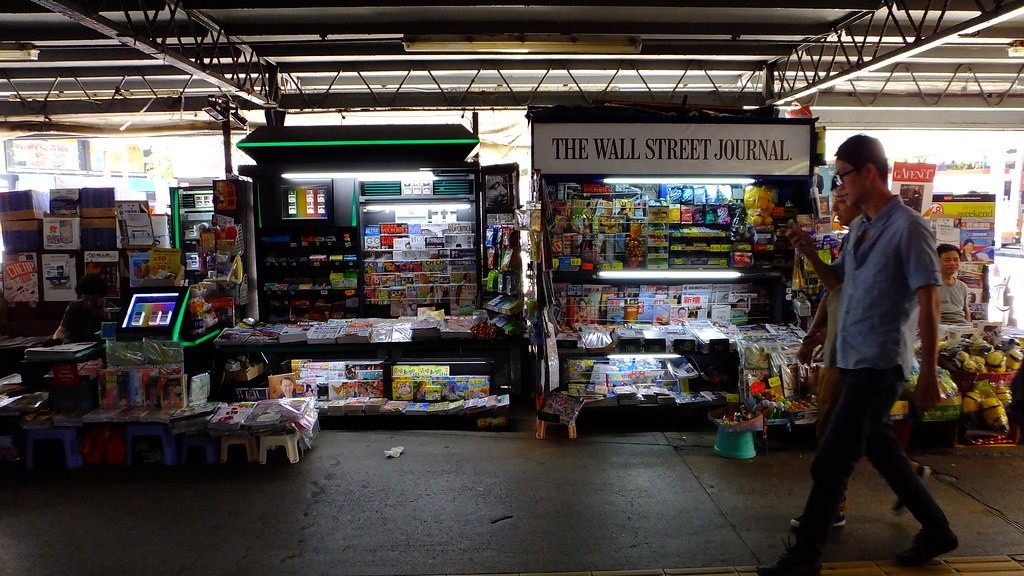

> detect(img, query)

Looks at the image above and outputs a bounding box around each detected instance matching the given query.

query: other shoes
[891,465,931,516]
[790,508,846,526]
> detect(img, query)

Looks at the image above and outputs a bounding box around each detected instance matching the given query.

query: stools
[220,431,259,465]
[259,434,301,465]
[535,414,578,439]
[126,425,176,466]
[180,436,215,464]
[24,427,80,468]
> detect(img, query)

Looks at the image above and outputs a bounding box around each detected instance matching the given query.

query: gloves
[42,338,64,347]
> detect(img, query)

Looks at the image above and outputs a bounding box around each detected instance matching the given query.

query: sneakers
[756,533,822,576]
[896,528,959,563]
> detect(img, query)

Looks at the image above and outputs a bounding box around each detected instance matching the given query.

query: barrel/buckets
[716,429,757,458]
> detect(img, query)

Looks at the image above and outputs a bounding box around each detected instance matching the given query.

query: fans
[991,284,1018,326]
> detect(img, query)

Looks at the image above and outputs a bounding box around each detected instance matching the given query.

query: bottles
[747,376,791,419]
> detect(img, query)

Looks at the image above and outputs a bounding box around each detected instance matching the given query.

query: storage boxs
[918,394,961,422]
[0,187,116,250]
[262,234,358,320]
[890,399,909,420]
[948,367,1021,392]
[708,403,763,432]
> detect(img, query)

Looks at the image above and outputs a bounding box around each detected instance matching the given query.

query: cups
[59,220,73,244]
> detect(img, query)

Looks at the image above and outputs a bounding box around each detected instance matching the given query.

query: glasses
[835,167,856,186]
[81,295,101,303]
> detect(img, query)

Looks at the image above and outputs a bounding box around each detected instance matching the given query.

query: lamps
[229,105,249,129]
[202,94,232,124]
[593,270,744,279]
[402,33,642,53]
[1005,39,1024,58]
[0,43,40,61]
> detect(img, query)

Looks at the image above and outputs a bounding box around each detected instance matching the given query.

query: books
[337,322,374,343]
[308,326,340,344]
[280,329,308,343]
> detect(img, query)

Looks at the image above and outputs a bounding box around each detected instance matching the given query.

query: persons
[53,276,116,344]
[278,377,296,398]
[678,307,686,318]
[757,134,958,576]
[427,287,435,303]
[456,285,466,306]
[936,244,971,323]
[305,384,313,397]
[462,273,471,283]
[960,239,978,261]
[487,177,507,206]
[605,217,622,233]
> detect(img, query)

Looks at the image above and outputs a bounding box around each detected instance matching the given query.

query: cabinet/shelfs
[556,348,738,434]
[213,335,518,431]
[141,265,186,286]
